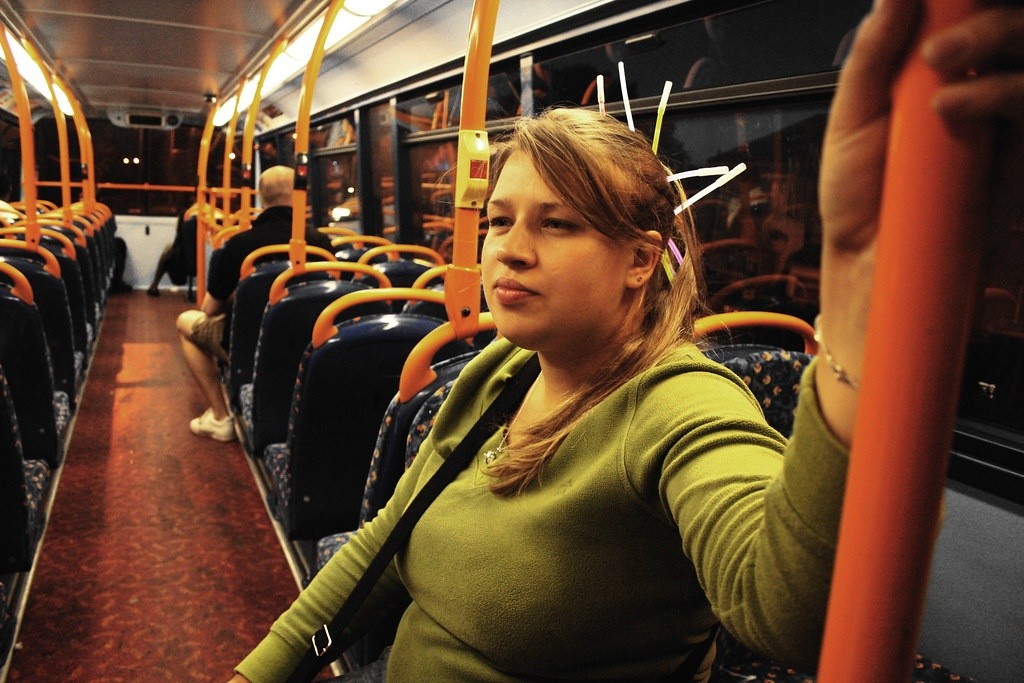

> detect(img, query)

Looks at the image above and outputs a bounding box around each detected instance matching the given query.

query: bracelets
[813,314,861,392]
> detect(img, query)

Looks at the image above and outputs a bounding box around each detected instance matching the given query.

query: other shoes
[146,287,160,296]
[109,282,132,295]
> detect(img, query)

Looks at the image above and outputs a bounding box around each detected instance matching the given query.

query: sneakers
[188,407,236,441]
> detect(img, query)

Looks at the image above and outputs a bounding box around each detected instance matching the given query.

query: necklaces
[485,370,592,467]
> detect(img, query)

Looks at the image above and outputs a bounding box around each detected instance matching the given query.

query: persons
[227,2,1024,683]
[146,190,223,298]
[176,166,341,442]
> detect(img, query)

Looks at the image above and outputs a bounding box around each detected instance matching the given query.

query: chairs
[0,199,116,670]
[211,198,819,580]
